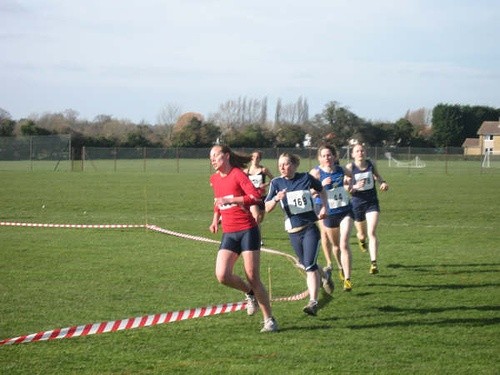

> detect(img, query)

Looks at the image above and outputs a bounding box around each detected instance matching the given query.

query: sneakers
[344,278,352,292]
[259,316,278,334]
[322,267,334,294]
[245,292,258,315]
[339,268,345,280]
[357,233,368,252]
[302,300,318,317]
[369,263,378,274]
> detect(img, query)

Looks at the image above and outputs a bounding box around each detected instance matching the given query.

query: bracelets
[381,180,385,184]
[272,196,278,203]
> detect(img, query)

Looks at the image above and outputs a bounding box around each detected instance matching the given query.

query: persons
[209,144,277,334]
[264,152,334,317]
[343,144,389,274]
[307,144,355,292]
[241,151,276,246]
